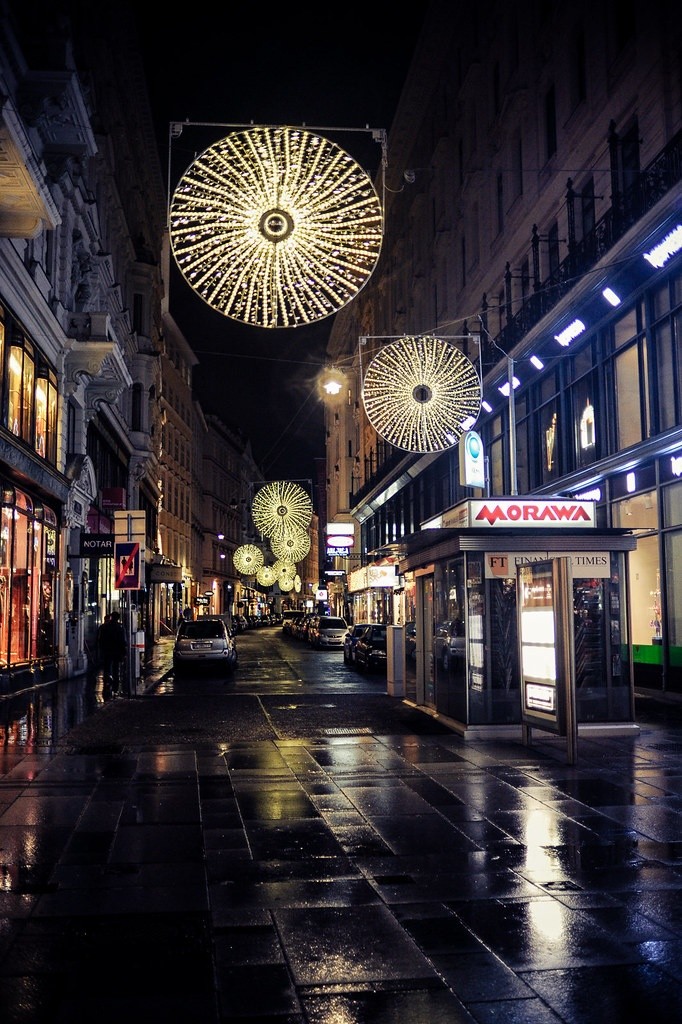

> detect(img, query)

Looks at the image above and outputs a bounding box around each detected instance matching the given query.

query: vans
[283,610,302,628]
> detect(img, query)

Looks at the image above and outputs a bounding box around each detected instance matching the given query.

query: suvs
[174,617,238,666]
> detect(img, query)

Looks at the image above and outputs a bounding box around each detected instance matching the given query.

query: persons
[95,611,126,700]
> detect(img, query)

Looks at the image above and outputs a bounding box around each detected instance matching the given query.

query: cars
[404,620,469,671]
[232,612,284,632]
[289,613,353,648]
[343,624,390,674]
